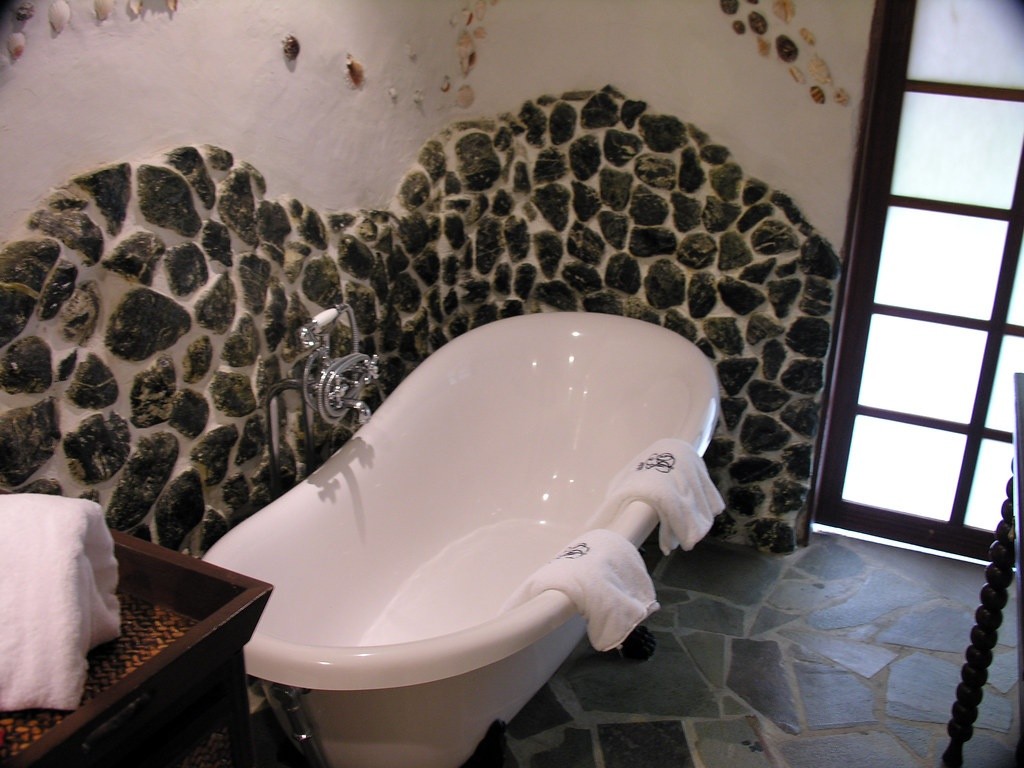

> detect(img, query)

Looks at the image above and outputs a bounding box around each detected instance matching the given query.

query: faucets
[304,390,375,433]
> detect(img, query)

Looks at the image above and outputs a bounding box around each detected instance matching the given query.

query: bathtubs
[194,309,724,767]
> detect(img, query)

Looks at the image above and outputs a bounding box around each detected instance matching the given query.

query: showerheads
[279,310,343,369]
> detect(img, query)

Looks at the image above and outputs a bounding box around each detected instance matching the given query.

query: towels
[576,437,732,559]
[501,530,663,652]
[0,483,123,718]
